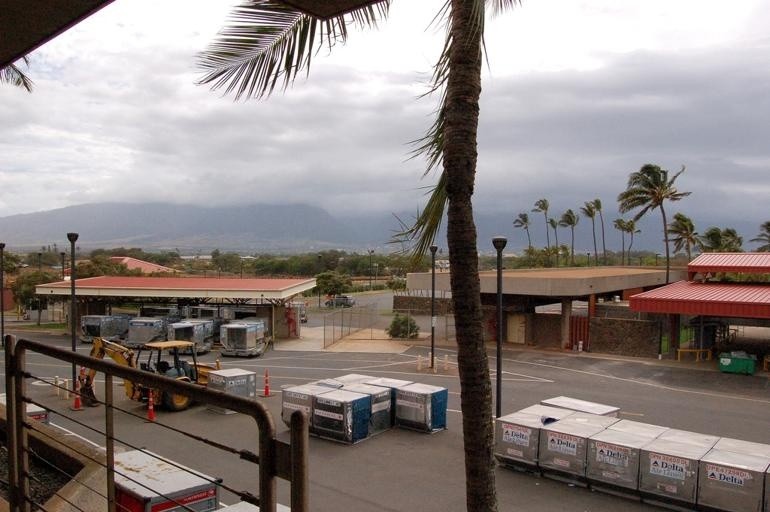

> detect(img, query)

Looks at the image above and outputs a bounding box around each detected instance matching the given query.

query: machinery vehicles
[76,333,224,416]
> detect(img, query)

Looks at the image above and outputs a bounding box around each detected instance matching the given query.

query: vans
[324,294,355,308]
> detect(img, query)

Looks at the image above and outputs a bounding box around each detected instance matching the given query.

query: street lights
[0,241,7,348]
[367,249,375,289]
[35,298,41,327]
[37,252,42,273]
[655,252,661,266]
[65,231,81,392]
[426,243,440,371]
[59,252,67,318]
[24,298,33,315]
[317,253,323,309]
[490,233,510,419]
[587,251,591,266]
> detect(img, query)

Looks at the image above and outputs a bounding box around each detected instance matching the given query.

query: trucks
[289,302,308,323]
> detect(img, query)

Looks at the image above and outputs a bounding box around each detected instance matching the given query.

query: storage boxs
[493,395,770,512]
[113,448,223,512]
[281,373,448,445]
[0,392,50,423]
[206,368,256,414]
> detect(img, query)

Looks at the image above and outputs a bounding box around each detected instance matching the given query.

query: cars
[17,263,29,268]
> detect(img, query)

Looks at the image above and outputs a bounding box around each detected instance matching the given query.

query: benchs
[676,349,712,362]
[763,356,770,369]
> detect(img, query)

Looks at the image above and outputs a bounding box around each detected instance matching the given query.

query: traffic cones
[70,395,84,412]
[71,380,81,397]
